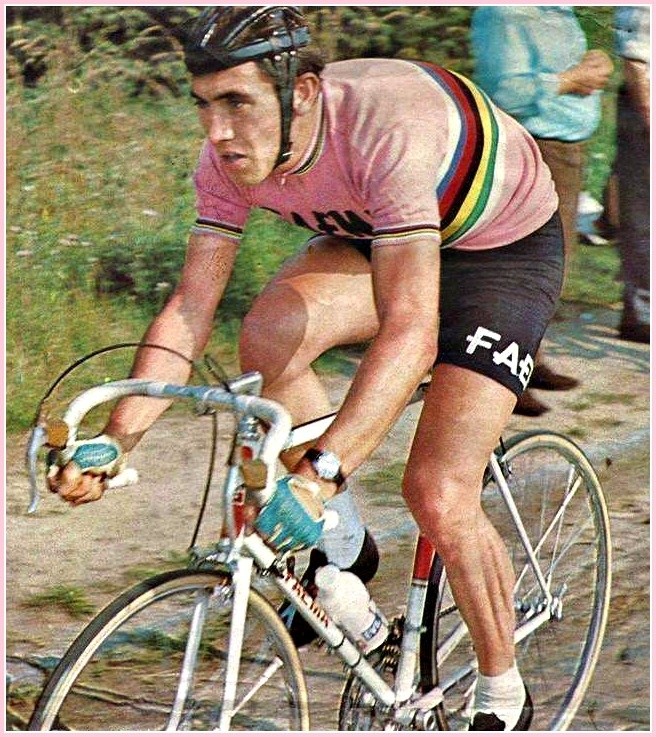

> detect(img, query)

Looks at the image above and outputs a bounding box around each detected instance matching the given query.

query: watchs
[302,447,346,496]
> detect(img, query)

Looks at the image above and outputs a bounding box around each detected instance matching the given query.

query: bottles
[315,564,390,655]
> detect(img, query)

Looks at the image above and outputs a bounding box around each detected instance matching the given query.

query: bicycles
[23,341,614,732]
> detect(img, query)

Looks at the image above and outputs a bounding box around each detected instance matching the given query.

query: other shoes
[527,363,579,391]
[512,389,549,417]
[576,215,622,247]
[617,315,649,341]
[466,679,533,731]
[277,527,380,650]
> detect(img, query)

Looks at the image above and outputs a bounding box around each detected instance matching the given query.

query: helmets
[171,6,311,75]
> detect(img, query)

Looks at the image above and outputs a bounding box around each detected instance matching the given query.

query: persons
[464,8,650,417]
[45,6,569,731]
